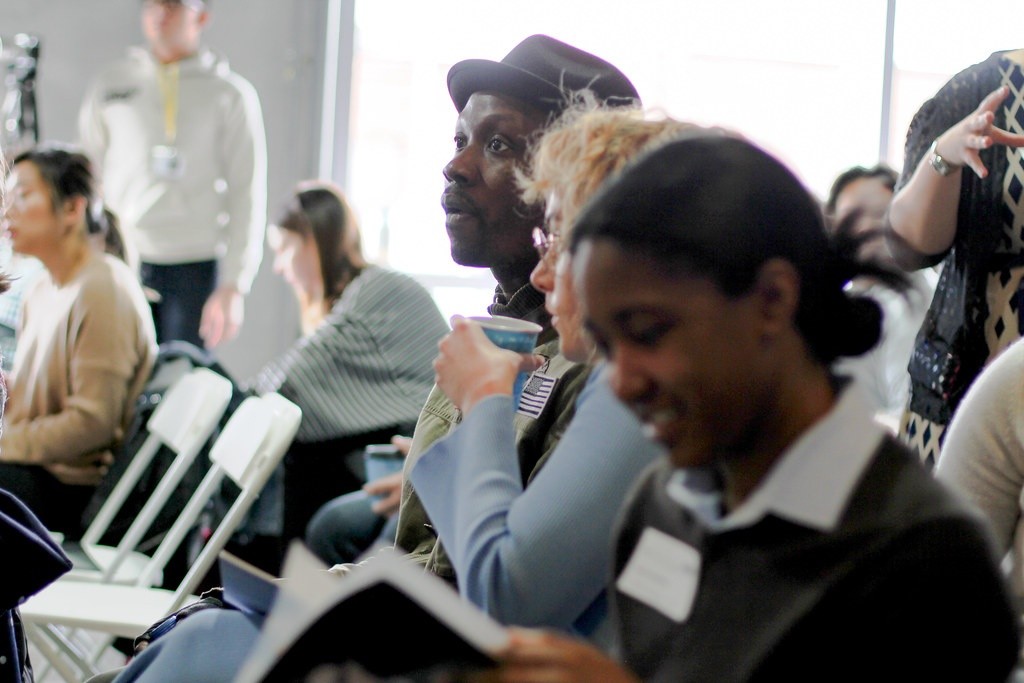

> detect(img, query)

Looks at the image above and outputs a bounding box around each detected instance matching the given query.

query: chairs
[15,392,303,683]
[44,365,233,587]
[72,340,235,546]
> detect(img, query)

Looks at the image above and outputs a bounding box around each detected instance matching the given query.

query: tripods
[1,81,39,143]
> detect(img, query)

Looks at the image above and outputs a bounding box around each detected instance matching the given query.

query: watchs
[930,137,962,176]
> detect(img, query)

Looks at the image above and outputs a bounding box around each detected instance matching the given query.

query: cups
[461,315,545,410]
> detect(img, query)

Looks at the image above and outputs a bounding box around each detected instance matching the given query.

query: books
[232,539,508,683]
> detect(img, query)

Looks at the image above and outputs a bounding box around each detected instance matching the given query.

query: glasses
[532,225,565,256]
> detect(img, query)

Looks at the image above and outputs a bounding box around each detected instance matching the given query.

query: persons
[0,0,1024,683]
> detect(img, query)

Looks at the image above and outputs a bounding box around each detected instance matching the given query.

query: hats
[447,34,642,114]
